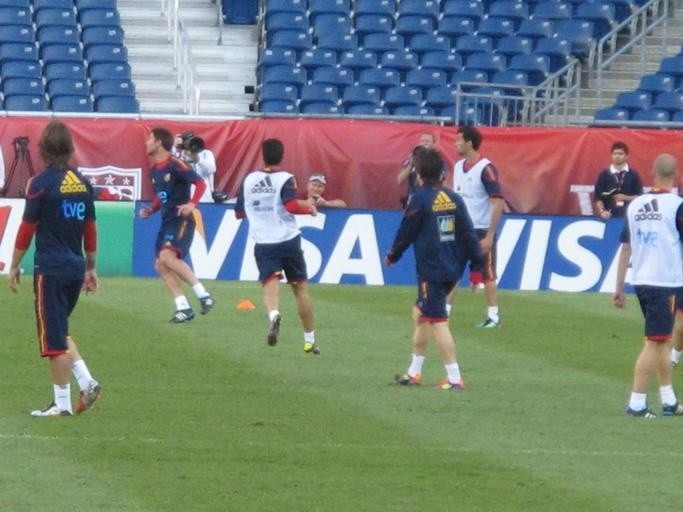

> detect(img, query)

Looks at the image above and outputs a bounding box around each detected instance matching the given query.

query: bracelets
[598,208,606,214]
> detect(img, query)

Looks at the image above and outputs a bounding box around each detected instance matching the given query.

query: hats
[309,174,326,185]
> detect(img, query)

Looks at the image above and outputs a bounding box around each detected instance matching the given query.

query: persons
[612,152,683,418]
[395,132,451,210]
[139,129,216,324]
[171,129,216,204]
[594,140,644,220]
[234,140,320,355]
[669,288,683,370]
[7,120,101,417]
[384,148,487,391]
[296,174,347,209]
[444,126,506,328]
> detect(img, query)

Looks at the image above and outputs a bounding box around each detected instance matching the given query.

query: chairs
[0,0,142,114]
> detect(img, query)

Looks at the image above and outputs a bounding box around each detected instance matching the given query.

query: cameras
[174,132,205,153]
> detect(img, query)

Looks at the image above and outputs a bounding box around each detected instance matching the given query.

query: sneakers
[303,341,320,354]
[168,307,195,323]
[626,405,658,419]
[661,400,683,416]
[75,378,101,414]
[267,313,282,345]
[387,372,421,386]
[475,316,501,329]
[671,361,679,369]
[30,400,73,418]
[432,377,464,391]
[199,296,214,315]
